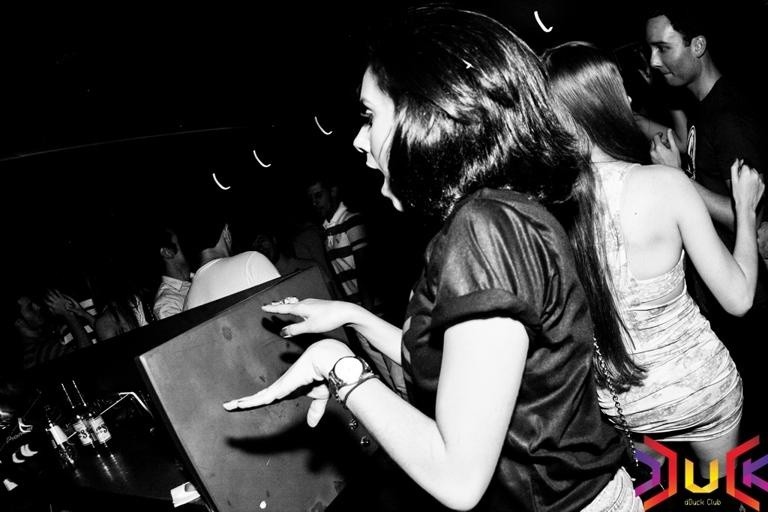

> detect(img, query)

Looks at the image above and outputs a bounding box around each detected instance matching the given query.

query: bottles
[43,379,119,471]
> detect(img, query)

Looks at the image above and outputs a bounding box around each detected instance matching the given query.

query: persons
[2,0,768,509]
